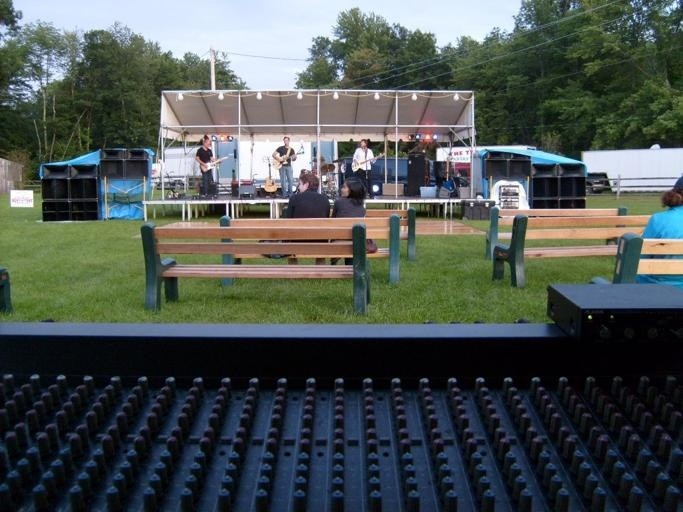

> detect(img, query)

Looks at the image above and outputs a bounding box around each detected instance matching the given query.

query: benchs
[139,207,416,316]
[484,208,682,288]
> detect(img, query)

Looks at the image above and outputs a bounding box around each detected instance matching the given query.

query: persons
[352,139,378,199]
[194,138,223,199]
[271,136,297,199]
[432,161,452,199]
[283,173,331,243]
[631,175,682,286]
[328,176,367,243]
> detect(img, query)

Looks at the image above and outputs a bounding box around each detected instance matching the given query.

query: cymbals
[322,164,335,172]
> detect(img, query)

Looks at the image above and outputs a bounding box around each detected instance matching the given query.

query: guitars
[443,158,456,193]
[201,157,229,172]
[351,153,384,173]
[264,157,277,192]
[272,154,297,169]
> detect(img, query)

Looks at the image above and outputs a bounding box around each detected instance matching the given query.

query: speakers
[407,156,425,197]
[236,184,257,198]
